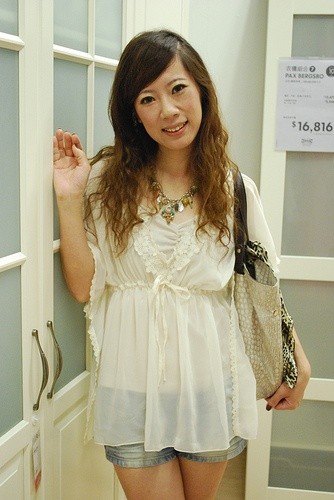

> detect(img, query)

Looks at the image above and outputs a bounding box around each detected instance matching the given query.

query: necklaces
[147,158,201,227]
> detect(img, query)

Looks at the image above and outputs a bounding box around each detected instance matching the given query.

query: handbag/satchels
[227,169,299,397]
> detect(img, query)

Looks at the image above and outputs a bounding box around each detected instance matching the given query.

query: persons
[50,28,312,500]
